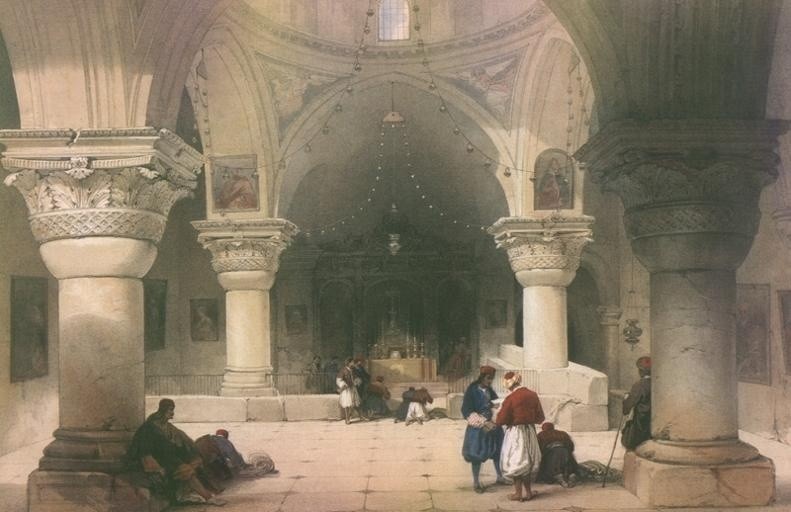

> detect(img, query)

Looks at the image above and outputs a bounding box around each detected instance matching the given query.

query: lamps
[624,249,642,352]
[384,113,407,259]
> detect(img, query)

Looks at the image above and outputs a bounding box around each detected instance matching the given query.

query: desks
[363,358,437,383]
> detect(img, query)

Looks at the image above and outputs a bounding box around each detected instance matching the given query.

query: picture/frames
[735,283,771,386]
[534,148,574,211]
[210,152,259,214]
[142,278,166,353]
[10,275,50,384]
[777,289,791,376]
[190,298,219,342]
[286,303,308,335]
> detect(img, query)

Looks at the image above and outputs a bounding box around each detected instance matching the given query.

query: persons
[535,157,569,209]
[536,422,577,489]
[194,429,254,480]
[303,354,435,426]
[210,162,256,210]
[482,370,545,501]
[620,356,652,449]
[460,365,512,494]
[129,397,230,507]
